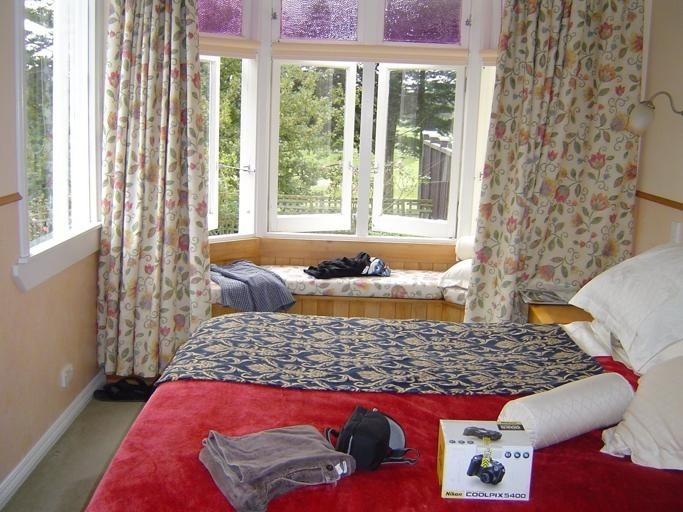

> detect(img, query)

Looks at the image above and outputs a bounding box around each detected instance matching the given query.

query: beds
[83,312,683,512]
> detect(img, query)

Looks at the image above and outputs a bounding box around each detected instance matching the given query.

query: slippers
[103,375,150,391]
[94,388,151,403]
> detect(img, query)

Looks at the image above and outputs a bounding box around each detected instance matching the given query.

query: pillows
[437,257,473,289]
[557,238,683,472]
[497,372,634,450]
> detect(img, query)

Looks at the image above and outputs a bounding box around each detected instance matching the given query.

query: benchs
[208,263,468,324]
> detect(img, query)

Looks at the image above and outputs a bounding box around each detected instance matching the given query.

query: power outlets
[60,363,75,388]
[670,221,683,245]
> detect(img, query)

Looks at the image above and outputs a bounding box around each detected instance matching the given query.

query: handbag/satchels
[324,405,420,472]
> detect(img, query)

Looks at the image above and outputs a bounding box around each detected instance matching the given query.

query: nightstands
[526,304,593,324]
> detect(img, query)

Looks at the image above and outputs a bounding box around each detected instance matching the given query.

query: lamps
[625,91,683,138]
